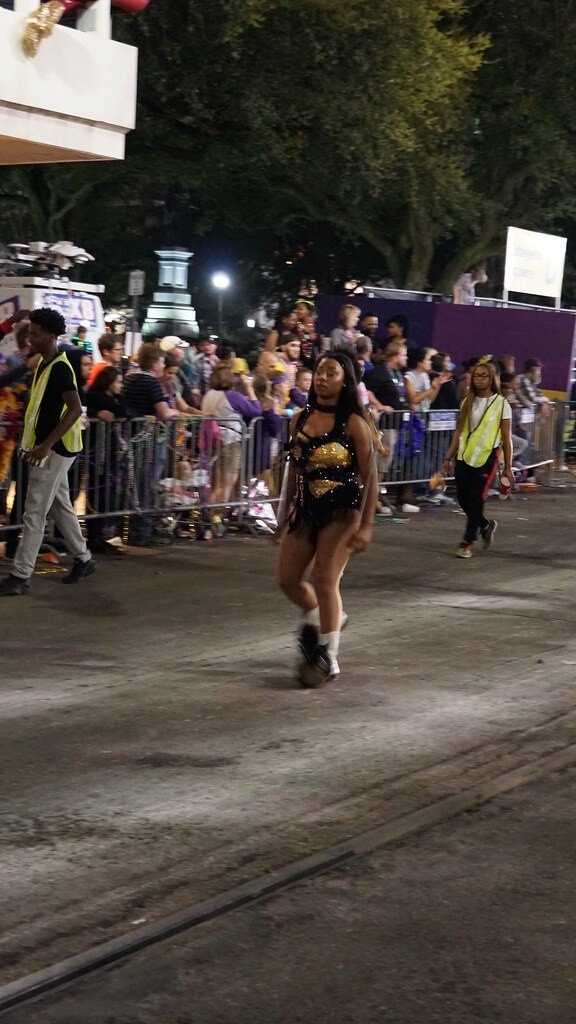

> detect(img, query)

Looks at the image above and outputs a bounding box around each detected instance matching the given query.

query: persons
[442,362,516,559]
[452,266,487,305]
[273,351,376,686]
[0,308,98,595]
[0,292,551,555]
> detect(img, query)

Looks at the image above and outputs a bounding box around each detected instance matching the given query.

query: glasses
[473,372,490,377]
[110,347,124,351]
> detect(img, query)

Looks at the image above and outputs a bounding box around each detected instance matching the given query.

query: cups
[400,503,420,513]
[499,477,510,500]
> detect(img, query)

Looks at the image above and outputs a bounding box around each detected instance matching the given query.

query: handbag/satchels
[200,415,220,456]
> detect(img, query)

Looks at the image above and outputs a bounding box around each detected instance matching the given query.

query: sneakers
[456,541,473,558]
[62,557,97,583]
[479,519,498,549]
[0,575,30,595]
[375,500,393,516]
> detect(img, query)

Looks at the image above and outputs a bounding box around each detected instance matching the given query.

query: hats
[160,336,190,351]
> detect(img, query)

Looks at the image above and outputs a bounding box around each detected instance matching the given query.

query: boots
[297,606,349,687]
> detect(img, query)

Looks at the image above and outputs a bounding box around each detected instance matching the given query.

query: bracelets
[444,459,450,461]
